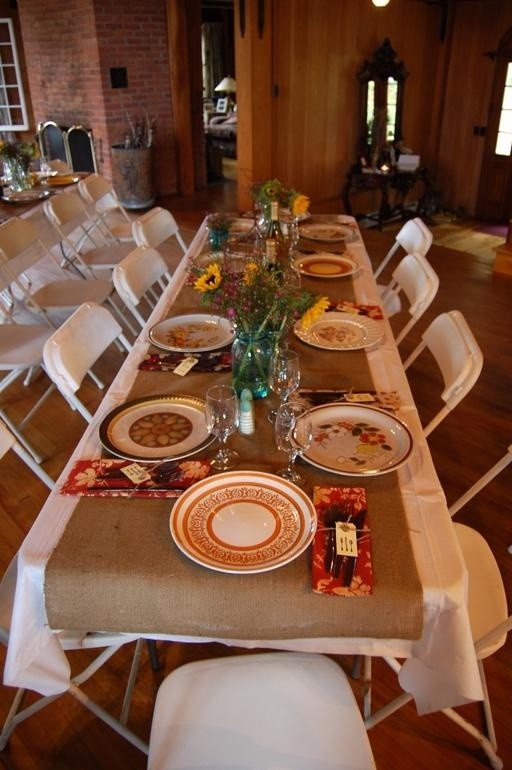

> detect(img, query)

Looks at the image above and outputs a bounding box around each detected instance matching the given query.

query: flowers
[237,178,312,229]
[184,253,310,329]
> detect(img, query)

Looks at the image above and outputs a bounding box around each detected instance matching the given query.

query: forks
[334,503,354,580]
[96,472,178,483]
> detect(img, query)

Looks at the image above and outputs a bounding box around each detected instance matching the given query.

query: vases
[227,333,280,404]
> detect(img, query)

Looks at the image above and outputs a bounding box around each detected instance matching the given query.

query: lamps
[213,75,236,112]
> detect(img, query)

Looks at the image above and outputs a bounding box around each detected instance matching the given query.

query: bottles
[263,201,285,289]
[239,388,255,436]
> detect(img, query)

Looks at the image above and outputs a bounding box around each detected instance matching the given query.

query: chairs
[141,651,380,770]
[113,243,173,329]
[1,420,152,760]
[40,299,131,425]
[203,116,237,182]
[0,172,134,498]
[382,251,440,349]
[374,215,434,300]
[130,203,188,277]
[403,308,485,441]
[361,440,512,769]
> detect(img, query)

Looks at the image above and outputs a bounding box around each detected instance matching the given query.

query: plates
[170,471,318,573]
[97,393,219,459]
[193,217,259,274]
[147,313,240,352]
[1,175,80,205]
[293,402,413,474]
[290,222,385,350]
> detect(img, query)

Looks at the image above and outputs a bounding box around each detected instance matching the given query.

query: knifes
[88,487,185,491]
[343,509,366,588]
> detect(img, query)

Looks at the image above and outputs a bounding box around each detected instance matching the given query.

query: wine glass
[206,385,241,471]
[267,350,312,422]
[274,403,313,488]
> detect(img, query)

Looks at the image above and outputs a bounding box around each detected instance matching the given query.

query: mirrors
[356,37,410,162]
